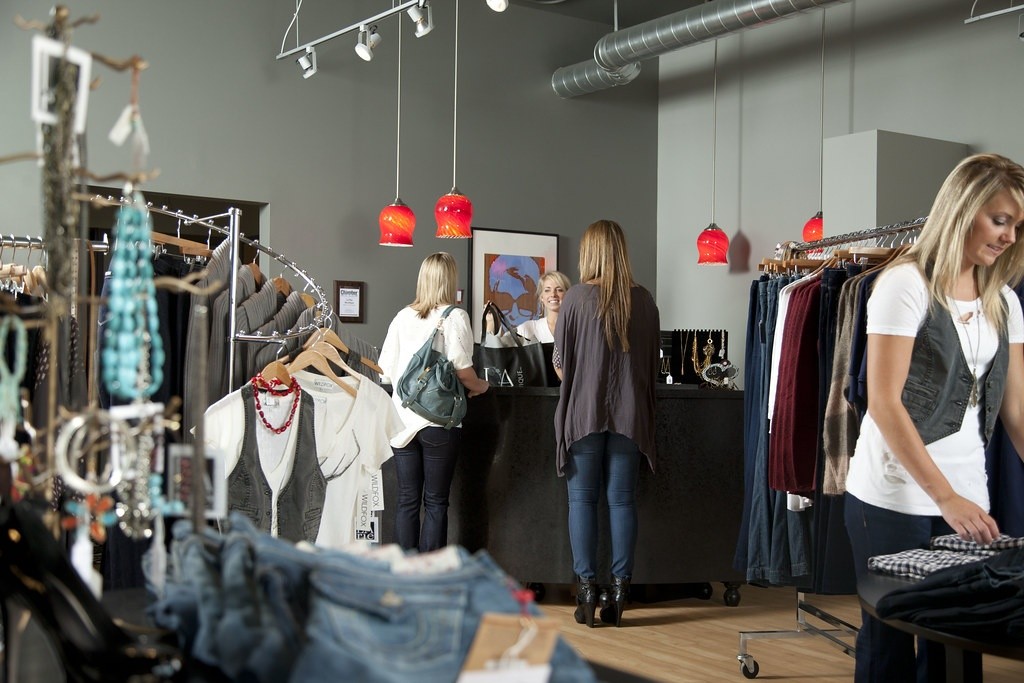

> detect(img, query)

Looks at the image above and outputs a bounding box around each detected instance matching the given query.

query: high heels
[600,574,629,626]
[573,576,598,628]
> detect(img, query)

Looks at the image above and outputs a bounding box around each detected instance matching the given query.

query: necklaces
[950,275,980,406]
[100,189,166,399]
[251,371,301,434]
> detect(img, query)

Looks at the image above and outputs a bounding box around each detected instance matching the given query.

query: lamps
[407,5,436,39]
[354,25,382,62]
[697,38,731,267]
[485,0,509,12]
[378,0,418,248]
[298,51,318,79]
[802,8,827,254]
[434,0,474,239]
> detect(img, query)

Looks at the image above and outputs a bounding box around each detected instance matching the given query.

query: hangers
[756,215,929,295]
[0,212,383,398]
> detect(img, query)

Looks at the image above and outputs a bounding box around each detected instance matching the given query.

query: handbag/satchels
[472,302,561,387]
[396,304,469,430]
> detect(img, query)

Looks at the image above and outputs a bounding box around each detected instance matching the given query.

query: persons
[843,151,1023,682]
[378,250,489,552]
[482,270,570,348]
[552,219,660,627]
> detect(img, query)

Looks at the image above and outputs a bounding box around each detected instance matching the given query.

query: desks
[857,570,1024,683]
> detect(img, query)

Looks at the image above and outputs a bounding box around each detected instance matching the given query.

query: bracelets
[49,409,155,542]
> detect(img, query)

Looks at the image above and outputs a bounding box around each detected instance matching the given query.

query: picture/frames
[332,279,365,324]
[467,227,560,343]
